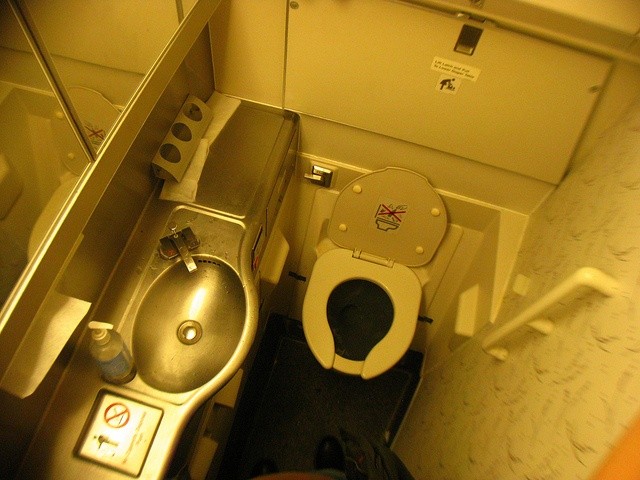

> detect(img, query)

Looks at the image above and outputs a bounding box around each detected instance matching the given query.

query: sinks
[129,252,247,394]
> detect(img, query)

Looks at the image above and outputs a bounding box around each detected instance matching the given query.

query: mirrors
[6,1,203,161]
[0,0,94,335]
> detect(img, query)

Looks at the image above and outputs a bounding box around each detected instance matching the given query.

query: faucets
[167,233,199,275]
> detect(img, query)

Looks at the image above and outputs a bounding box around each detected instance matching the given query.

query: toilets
[301,165,450,380]
[26,85,122,270]
